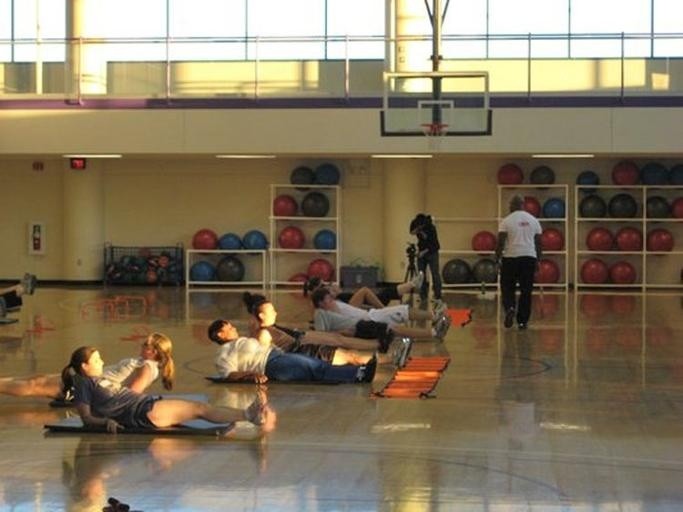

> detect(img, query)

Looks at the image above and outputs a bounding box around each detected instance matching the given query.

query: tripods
[404,264,417,283]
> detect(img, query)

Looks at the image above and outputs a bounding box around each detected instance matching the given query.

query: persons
[0,330,175,400]
[490,192,544,332]
[61,346,275,435]
[207,379,362,421]
[407,213,443,300]
[59,425,270,512]
[495,323,548,453]
[207,269,452,386]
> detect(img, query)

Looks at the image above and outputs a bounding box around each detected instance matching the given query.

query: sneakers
[414,270,424,289]
[395,337,413,367]
[433,299,449,340]
[503,307,528,329]
[361,353,377,383]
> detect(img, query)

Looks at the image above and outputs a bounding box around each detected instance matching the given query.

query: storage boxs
[340,266,377,288]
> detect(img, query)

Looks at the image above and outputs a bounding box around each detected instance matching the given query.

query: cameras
[407,244,415,254]
